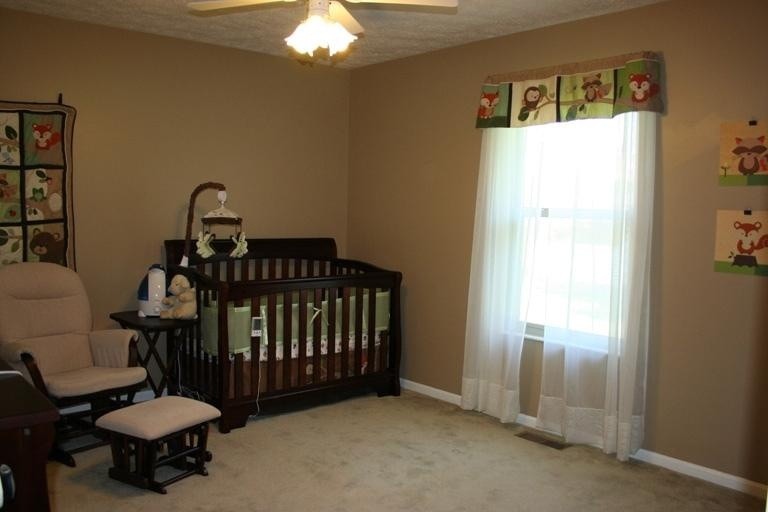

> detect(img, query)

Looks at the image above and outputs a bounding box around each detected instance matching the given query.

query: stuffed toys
[160,274,198,320]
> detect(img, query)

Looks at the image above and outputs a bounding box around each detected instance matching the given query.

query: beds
[165,235,403,435]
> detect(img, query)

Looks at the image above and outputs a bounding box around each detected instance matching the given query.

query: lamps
[283,0,359,59]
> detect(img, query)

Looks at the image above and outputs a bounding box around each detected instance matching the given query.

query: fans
[186,0,458,37]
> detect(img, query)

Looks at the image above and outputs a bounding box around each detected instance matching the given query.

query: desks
[1,356,61,512]
[109,309,199,398]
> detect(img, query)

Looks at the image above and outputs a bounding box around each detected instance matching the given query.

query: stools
[95,395,223,495]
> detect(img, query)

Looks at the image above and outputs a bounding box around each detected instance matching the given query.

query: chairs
[1,261,148,469]
